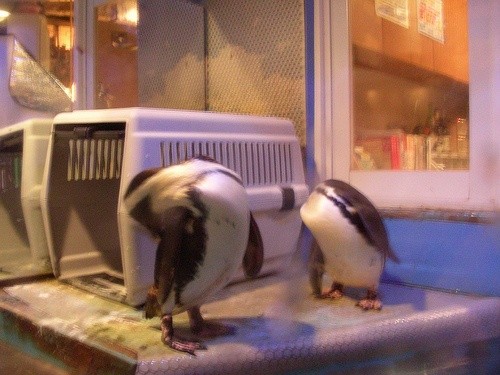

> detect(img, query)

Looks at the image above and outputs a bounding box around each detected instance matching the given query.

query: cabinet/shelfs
[347,0,468,96]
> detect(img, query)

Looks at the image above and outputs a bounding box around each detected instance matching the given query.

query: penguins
[123,154,265,356]
[299,179,401,312]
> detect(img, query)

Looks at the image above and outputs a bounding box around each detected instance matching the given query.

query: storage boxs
[0,106,309,306]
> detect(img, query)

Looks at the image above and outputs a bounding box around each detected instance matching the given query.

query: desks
[0,262,500,375]
